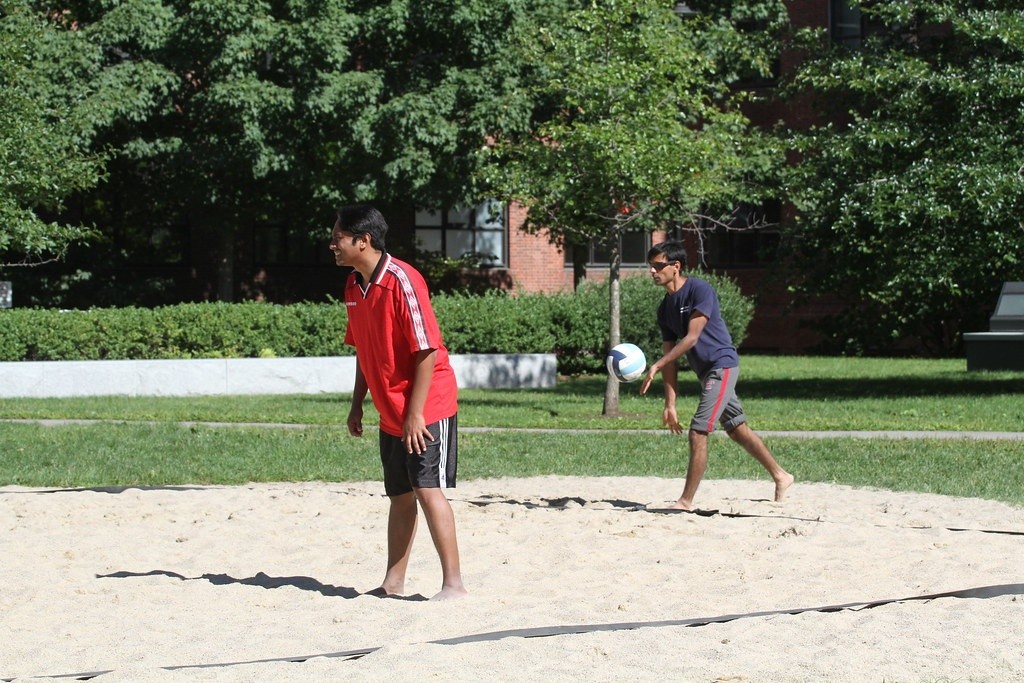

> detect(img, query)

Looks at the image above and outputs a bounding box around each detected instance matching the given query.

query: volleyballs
[606,343,648,383]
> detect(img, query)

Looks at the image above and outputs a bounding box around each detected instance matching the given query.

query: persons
[639,241,794,509]
[328,205,465,600]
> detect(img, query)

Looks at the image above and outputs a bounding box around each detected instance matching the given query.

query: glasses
[649,260,675,273]
